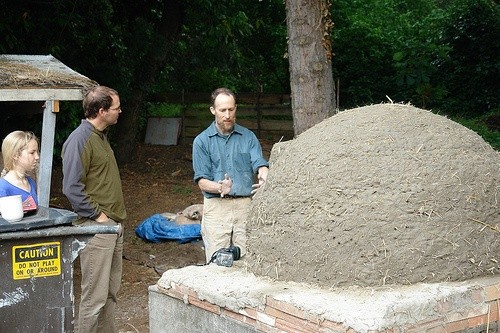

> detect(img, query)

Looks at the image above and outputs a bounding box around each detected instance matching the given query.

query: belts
[222,194,245,198]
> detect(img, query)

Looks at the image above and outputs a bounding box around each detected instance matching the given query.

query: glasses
[105,106,121,110]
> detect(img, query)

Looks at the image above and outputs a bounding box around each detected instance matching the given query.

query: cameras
[216,246,241,267]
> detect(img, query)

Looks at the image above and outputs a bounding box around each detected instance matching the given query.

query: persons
[0,131,40,211]
[191,88,269,265]
[60,85,127,333]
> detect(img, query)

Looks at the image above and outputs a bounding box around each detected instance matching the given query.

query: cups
[0,194,24,224]
